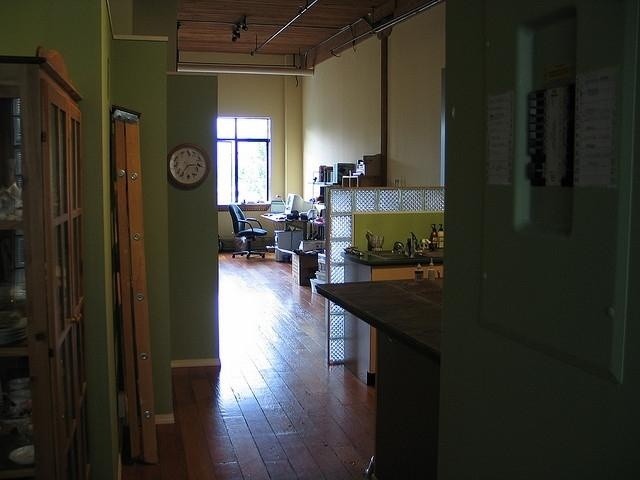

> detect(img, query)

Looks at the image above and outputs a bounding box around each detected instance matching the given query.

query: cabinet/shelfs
[0,45,92,480]
[305,171,384,205]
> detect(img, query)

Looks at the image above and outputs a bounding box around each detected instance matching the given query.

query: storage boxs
[278,247,318,285]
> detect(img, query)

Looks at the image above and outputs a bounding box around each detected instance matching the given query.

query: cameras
[287,210,300,220]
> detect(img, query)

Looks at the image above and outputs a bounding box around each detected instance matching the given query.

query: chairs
[227,203,267,261]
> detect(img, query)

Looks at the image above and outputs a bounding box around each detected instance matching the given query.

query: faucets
[408,231,422,259]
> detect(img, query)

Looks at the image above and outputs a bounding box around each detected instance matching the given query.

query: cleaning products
[429,223,438,250]
[437,223,445,248]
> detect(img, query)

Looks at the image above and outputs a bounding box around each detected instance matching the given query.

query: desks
[259,212,313,254]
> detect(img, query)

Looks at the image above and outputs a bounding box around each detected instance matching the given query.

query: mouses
[279,217,284,219]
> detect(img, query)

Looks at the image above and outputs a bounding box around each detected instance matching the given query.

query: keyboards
[275,215,286,219]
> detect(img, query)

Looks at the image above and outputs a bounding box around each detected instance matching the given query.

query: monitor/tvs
[285,194,313,214]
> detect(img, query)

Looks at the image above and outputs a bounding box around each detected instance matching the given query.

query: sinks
[368,250,410,261]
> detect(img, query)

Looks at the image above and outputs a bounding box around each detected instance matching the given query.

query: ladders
[110,104,159,463]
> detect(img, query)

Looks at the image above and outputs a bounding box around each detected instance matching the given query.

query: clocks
[168,142,211,190]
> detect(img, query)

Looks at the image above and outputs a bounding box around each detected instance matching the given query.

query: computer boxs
[274,230,303,262]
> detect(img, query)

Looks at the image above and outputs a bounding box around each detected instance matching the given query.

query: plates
[0,311,27,345]
[0,414,30,423]
[9,445,35,465]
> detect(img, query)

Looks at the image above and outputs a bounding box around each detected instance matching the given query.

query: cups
[7,377,31,417]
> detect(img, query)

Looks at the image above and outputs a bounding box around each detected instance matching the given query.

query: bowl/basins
[369,236,385,248]
